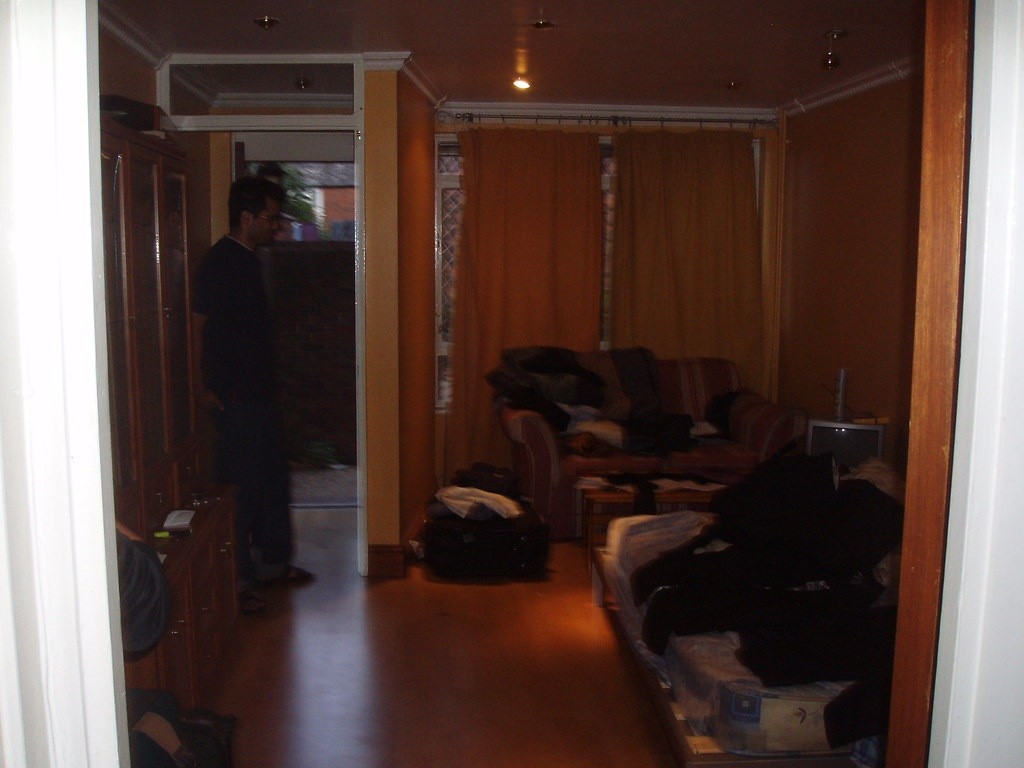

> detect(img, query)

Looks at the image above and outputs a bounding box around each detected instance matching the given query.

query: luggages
[424,513,550,578]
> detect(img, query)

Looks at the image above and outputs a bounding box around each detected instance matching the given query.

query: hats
[256,160,292,178]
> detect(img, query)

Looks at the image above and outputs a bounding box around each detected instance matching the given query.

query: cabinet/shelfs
[101,117,241,711]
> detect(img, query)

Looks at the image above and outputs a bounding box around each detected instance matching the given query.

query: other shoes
[249,563,313,583]
[239,589,268,614]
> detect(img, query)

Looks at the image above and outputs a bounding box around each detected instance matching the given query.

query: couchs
[496,356,808,539]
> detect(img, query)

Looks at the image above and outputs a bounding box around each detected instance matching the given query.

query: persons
[116,519,227,768]
[192,176,316,613]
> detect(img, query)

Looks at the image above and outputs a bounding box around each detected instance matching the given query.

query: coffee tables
[579,471,725,572]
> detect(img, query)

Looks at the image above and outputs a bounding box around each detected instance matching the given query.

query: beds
[593,510,905,768]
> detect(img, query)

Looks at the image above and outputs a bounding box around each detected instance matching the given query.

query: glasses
[245,207,282,225]
